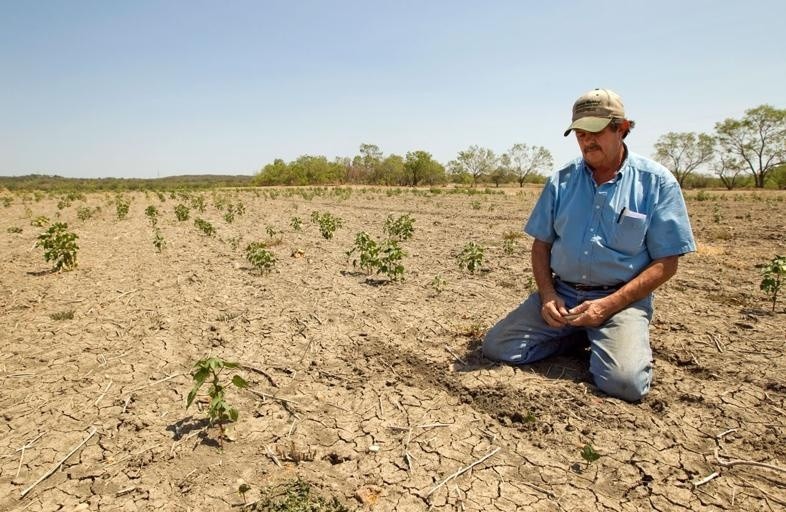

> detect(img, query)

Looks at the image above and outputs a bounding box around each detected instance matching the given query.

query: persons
[481,90,697,402]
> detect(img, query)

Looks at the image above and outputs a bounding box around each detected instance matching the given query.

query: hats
[565,88,625,136]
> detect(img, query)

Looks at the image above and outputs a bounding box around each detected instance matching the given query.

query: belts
[558,276,619,291]
